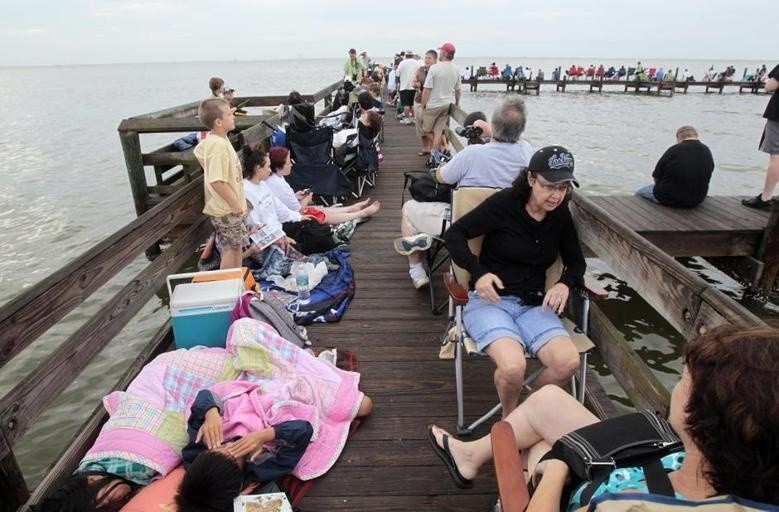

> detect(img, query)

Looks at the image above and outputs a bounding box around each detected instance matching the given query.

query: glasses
[534,176,571,191]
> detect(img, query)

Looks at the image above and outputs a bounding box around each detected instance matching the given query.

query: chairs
[443,186,597,434]
[425,207,451,314]
[283,101,384,206]
[587,492,777,511]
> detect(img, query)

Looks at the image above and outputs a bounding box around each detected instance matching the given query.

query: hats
[358,92,374,111]
[437,43,456,53]
[404,49,417,56]
[223,85,235,94]
[529,144,581,188]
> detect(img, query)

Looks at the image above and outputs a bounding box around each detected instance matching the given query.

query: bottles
[295,265,310,306]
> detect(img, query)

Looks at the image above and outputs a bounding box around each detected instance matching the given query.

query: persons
[193,45,384,271]
[443,146,588,424]
[49,316,373,512]
[463,62,767,85]
[388,44,463,170]
[741,63,779,207]
[395,99,537,290]
[425,318,778,511]
[633,126,715,207]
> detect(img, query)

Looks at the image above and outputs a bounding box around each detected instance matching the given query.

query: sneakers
[424,158,435,168]
[440,148,450,155]
[399,118,412,125]
[418,149,431,156]
[409,267,430,290]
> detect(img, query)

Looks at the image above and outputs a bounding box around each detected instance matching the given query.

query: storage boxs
[166,268,245,350]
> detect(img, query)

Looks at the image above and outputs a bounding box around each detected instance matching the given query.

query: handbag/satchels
[551,407,684,480]
[409,173,451,202]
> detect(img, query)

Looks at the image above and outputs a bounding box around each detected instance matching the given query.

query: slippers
[426,422,475,490]
